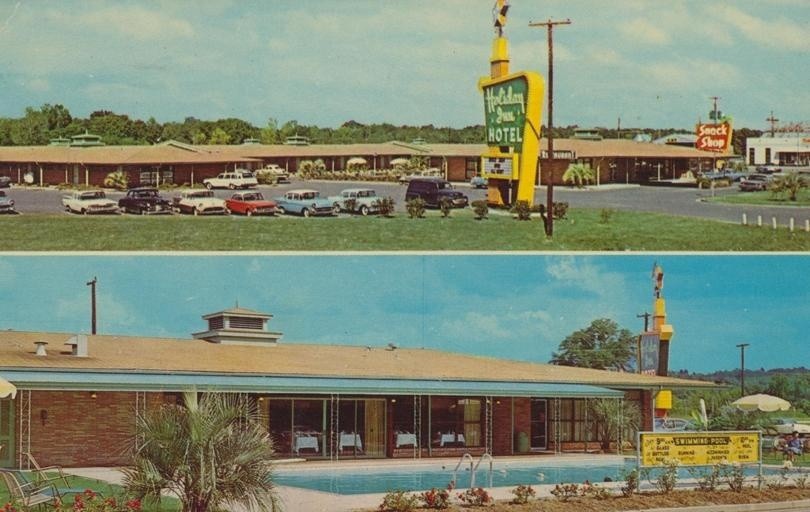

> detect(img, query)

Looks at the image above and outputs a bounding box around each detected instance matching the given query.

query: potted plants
[621,440,633,454]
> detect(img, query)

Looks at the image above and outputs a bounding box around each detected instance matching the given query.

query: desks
[293,433,319,457]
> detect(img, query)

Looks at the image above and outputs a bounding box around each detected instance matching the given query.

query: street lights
[736,343,749,396]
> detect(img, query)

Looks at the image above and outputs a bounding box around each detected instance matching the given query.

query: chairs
[782,438,810,462]
[773,435,787,460]
[0,472,56,512]
[21,452,76,489]
[15,471,106,512]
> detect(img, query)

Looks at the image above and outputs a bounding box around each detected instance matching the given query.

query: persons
[782,430,803,462]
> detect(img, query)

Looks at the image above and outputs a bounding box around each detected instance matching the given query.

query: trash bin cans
[517,431,530,454]
[24,172,35,186]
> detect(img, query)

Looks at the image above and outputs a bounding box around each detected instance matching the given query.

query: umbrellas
[729,393,792,413]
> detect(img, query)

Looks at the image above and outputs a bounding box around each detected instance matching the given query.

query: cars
[705,163,810,191]
[0,173,15,213]
[655,418,697,431]
[771,418,798,425]
